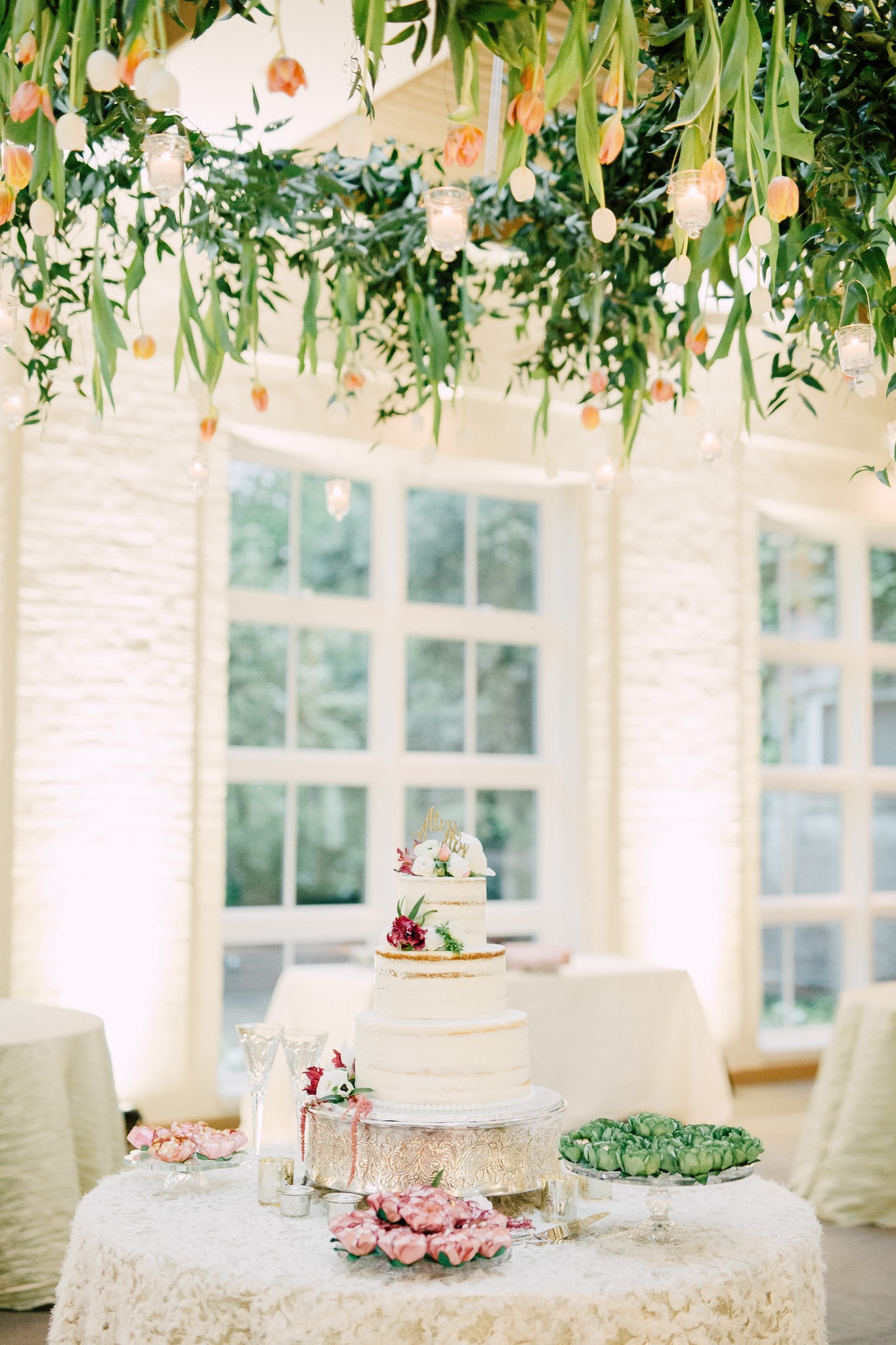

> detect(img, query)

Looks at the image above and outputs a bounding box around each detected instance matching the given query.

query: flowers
[397,803,498,882]
[386,891,465,956]
[298,1052,374,1190]
[125,1120,251,1165]
[557,1110,768,1185]
[327,1163,516,1271]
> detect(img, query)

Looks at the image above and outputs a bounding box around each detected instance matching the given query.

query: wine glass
[233,1023,284,1177]
[279,1029,328,1175]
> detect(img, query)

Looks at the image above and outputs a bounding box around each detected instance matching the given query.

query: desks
[777,979,896,1230]
[0,994,131,1314]
[246,952,737,1162]
[42,1139,833,1345]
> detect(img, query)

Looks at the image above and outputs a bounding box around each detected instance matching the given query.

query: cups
[541,1173,578,1224]
[578,1172,612,1201]
[323,1192,364,1222]
[276,1185,314,1218]
[258,1157,295,1207]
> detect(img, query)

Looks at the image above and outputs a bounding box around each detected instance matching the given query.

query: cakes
[350,805,528,1107]
